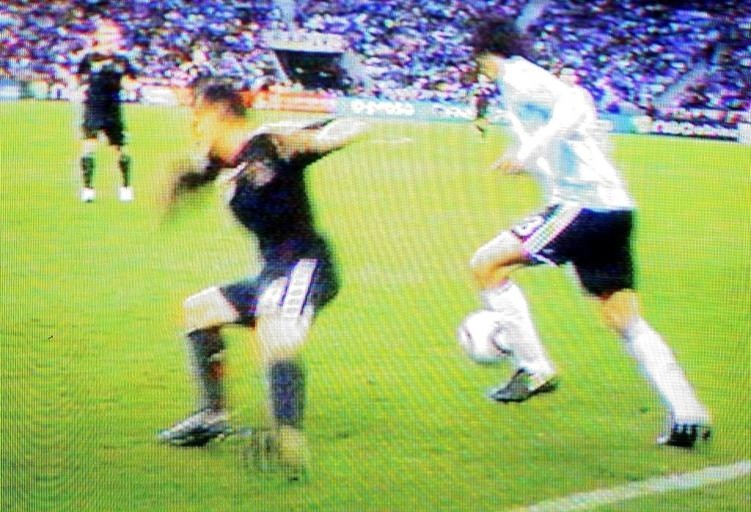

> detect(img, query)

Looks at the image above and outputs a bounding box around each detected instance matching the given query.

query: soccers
[459,311,516,364]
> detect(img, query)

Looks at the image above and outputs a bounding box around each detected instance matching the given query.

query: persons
[464,8,712,451]
[1,1,749,122]
[156,80,369,486]
[473,89,488,142]
[72,15,138,208]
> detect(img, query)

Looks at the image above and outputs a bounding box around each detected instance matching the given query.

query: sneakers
[120,184,135,204]
[656,420,712,449]
[81,187,94,206]
[490,365,561,403]
[156,411,234,450]
